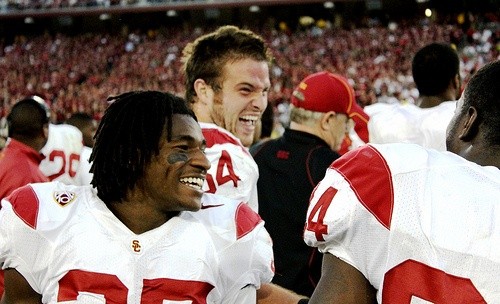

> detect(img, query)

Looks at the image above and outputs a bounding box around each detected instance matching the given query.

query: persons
[0,96,98,208]
[363,42,460,152]
[179,25,309,304]
[0,0,500,137]
[0,90,275,304]
[303,58,500,304]
[249,72,370,304]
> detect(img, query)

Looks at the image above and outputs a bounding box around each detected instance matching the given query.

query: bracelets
[297,299,310,304]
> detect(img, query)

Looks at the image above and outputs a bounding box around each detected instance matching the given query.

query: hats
[291,71,370,126]
